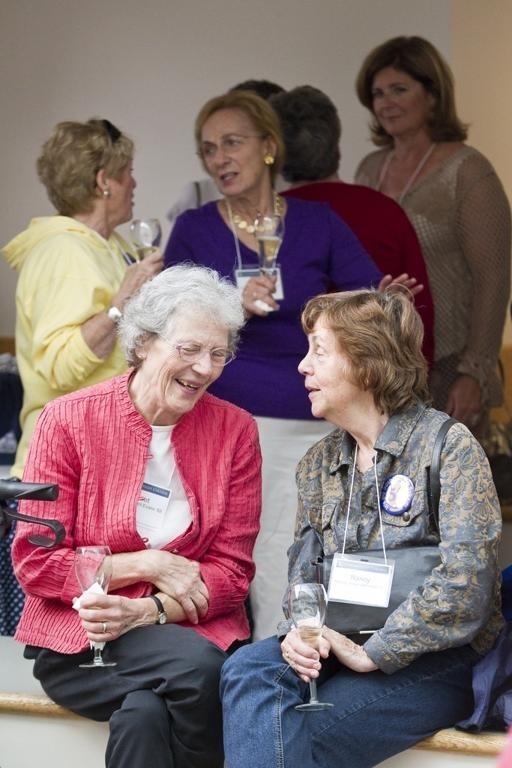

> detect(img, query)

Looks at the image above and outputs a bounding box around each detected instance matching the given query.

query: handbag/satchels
[312,546,441,633]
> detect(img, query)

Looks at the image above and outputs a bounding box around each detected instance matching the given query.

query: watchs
[150,596,168,625]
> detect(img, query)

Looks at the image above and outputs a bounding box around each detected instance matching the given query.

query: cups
[129,217,162,260]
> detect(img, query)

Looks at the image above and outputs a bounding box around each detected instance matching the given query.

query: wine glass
[253,214,286,311]
[290,584,336,711]
[75,545,118,667]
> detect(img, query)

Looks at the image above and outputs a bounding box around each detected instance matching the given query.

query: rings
[285,651,289,657]
[253,291,256,300]
[102,622,106,633]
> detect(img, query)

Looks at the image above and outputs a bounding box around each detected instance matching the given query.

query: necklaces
[220,190,283,234]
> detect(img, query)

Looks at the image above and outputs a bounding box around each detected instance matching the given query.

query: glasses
[157,333,237,367]
[102,119,120,142]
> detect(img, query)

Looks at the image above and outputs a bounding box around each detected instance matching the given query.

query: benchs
[0,653,512,767]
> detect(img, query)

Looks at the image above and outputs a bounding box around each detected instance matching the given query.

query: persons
[167,77,294,247]
[8,263,262,768]
[164,90,425,423]
[219,287,504,767]
[1,116,167,633]
[352,34,511,457]
[267,84,436,411]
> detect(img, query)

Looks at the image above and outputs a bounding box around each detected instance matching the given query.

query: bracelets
[105,304,124,323]
